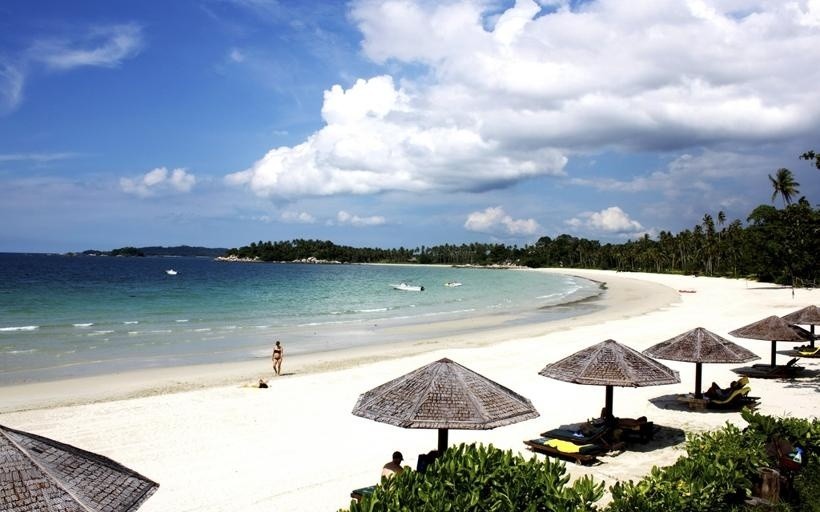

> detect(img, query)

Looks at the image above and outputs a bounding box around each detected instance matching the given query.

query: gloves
[165,269,178,275]
[388,282,425,292]
[444,280,463,288]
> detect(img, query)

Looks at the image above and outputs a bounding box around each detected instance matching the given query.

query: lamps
[709,377,754,407]
[524,419,655,464]
[734,357,801,379]
[798,345,820,356]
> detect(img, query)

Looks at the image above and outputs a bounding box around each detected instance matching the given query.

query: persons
[709,381,737,392]
[687,381,741,401]
[271,341,283,376]
[381,451,405,482]
[580,423,628,440]
[595,407,648,429]
